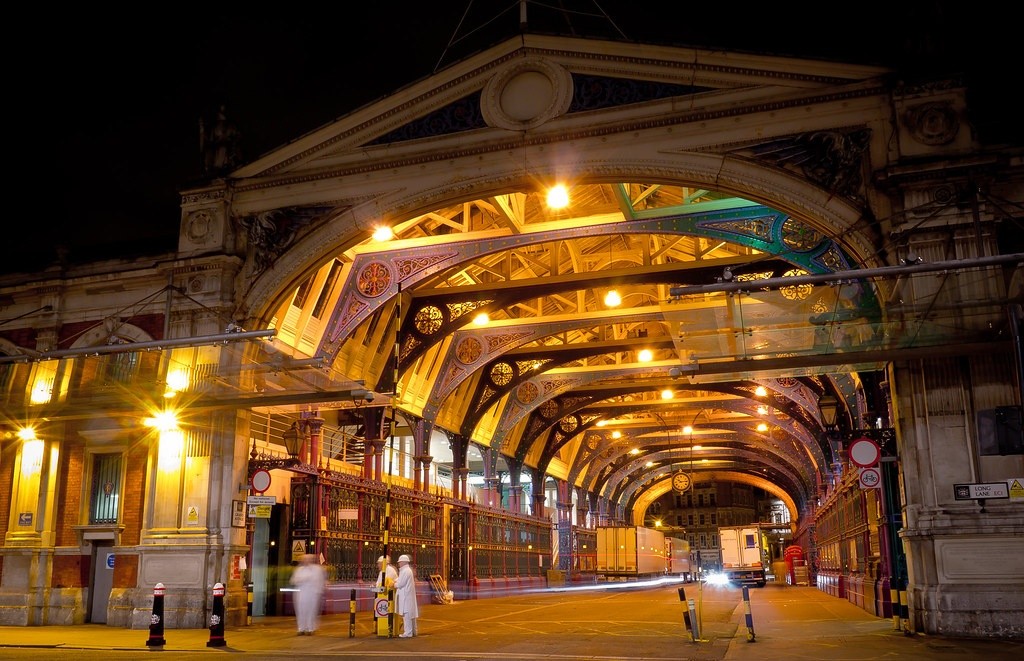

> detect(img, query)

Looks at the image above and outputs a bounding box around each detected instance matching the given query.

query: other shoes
[305,630,313,636]
[297,631,305,636]
[399,634,409,637]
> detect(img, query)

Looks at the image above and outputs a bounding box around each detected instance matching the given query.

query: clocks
[672,469,691,496]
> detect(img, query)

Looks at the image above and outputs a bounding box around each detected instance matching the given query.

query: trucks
[718,525,766,588]
[595,526,693,592]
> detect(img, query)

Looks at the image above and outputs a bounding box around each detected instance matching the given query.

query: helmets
[377,555,390,563]
[397,555,410,562]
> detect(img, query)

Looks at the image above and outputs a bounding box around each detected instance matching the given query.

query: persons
[394,555,419,637]
[290,553,327,635]
[376,555,398,587]
